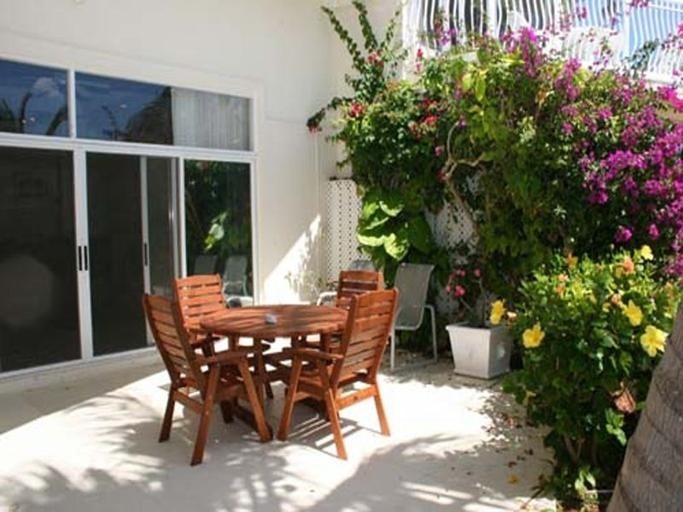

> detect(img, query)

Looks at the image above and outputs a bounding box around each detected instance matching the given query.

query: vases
[444,316,516,379]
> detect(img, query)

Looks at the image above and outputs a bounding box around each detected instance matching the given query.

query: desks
[199,304,348,431]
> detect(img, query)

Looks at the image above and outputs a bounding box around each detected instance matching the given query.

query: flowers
[445,265,492,328]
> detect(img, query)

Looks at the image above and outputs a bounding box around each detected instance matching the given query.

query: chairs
[140,292,272,464]
[192,253,252,305]
[170,272,276,422]
[382,265,440,370]
[276,288,399,460]
[282,270,383,410]
